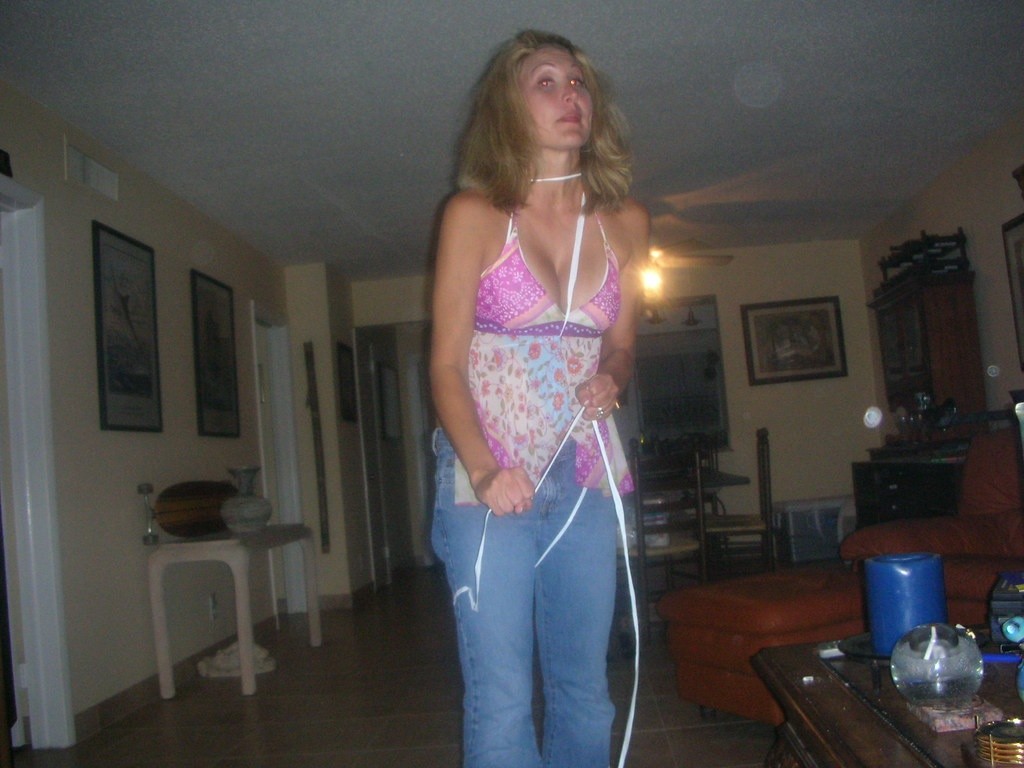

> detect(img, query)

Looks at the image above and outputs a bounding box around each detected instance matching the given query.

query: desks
[631,465,753,588]
[751,619,1024,768]
[146,522,324,699]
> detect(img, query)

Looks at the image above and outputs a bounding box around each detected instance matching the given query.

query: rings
[596,407,603,418]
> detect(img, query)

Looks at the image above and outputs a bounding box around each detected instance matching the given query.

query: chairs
[702,425,777,581]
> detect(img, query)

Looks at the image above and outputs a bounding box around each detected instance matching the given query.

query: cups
[909,415,924,441]
[896,413,909,440]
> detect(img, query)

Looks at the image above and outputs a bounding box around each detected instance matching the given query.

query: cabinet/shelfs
[865,268,988,409]
[851,455,969,534]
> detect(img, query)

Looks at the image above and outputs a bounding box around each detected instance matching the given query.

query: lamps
[682,309,701,328]
[646,305,666,325]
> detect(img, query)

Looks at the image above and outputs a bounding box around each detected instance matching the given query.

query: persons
[424,26,654,766]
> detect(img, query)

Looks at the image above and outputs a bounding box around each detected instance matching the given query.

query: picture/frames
[738,294,849,387]
[376,362,405,444]
[336,340,361,422]
[89,217,164,433]
[189,266,242,438]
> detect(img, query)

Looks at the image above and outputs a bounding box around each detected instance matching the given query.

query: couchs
[666,419,1024,736]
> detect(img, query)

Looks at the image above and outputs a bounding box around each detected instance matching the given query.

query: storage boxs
[771,493,847,566]
